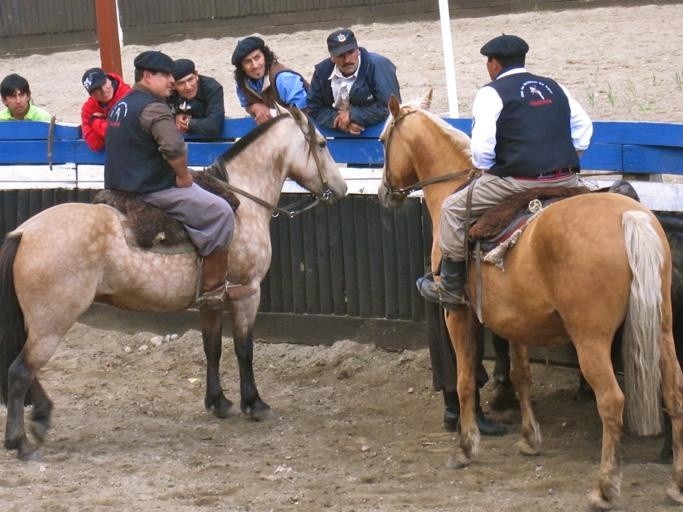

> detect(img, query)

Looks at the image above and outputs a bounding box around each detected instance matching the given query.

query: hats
[481,33,530,60]
[81,29,358,95]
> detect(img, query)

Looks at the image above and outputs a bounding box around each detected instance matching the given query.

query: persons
[168,57,224,139]
[0,74,52,125]
[81,66,132,153]
[103,50,258,311]
[421,195,507,437]
[412,35,594,310]
[307,29,401,135]
[231,36,310,126]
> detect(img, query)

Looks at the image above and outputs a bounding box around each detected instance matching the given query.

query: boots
[443,411,509,436]
[416,255,469,309]
[202,248,257,309]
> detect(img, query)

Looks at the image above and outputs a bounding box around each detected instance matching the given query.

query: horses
[378,87,683,512]
[0,99,348,463]
